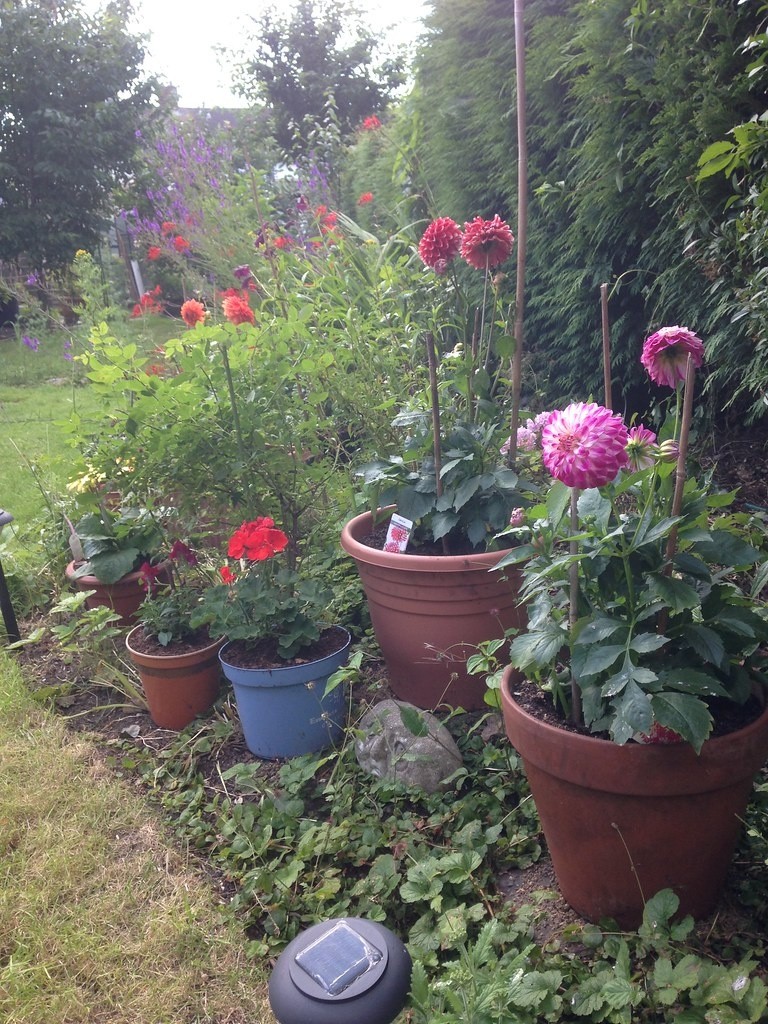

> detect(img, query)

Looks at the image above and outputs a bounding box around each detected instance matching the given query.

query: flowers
[43,117,767,758]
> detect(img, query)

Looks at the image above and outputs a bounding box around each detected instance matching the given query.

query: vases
[499,663,766,931]
[219,621,350,760]
[339,504,547,712]
[66,560,171,627]
[125,620,227,731]
